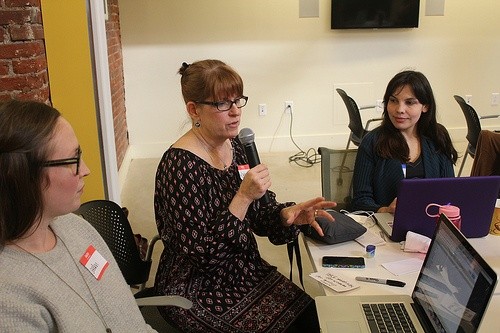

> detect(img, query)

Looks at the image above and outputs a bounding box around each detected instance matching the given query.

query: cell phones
[321,255,365,268]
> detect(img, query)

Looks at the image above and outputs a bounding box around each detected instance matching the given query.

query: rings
[314,210,318,217]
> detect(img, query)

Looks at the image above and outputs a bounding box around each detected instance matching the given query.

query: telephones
[322,256,365,268]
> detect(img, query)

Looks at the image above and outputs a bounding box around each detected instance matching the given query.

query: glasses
[199,95,248,111]
[41,144,82,176]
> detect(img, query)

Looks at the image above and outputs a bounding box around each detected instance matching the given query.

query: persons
[349,70,456,217]
[153,59,337,333]
[0,99,158,333]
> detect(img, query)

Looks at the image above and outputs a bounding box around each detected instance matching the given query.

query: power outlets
[465,95,472,104]
[285,101,295,114]
[259,104,267,115]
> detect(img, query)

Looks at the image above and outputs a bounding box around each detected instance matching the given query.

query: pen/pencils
[356,276,406,287]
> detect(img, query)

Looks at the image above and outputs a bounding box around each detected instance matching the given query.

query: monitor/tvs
[330,0,420,28]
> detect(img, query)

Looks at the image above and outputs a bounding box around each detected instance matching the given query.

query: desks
[300,219,500,333]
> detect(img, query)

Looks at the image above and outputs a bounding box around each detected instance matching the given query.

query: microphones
[238,128,271,207]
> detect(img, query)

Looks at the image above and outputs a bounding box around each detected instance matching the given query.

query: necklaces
[11,235,112,333]
[195,134,227,168]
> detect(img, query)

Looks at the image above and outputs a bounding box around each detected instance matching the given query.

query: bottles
[425,203,462,232]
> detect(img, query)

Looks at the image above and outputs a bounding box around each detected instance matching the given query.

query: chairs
[335,88,384,203]
[72,198,193,333]
[453,95,500,176]
[318,146,359,214]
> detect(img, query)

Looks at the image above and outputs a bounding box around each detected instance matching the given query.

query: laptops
[373,176,500,241]
[315,212,499,333]
